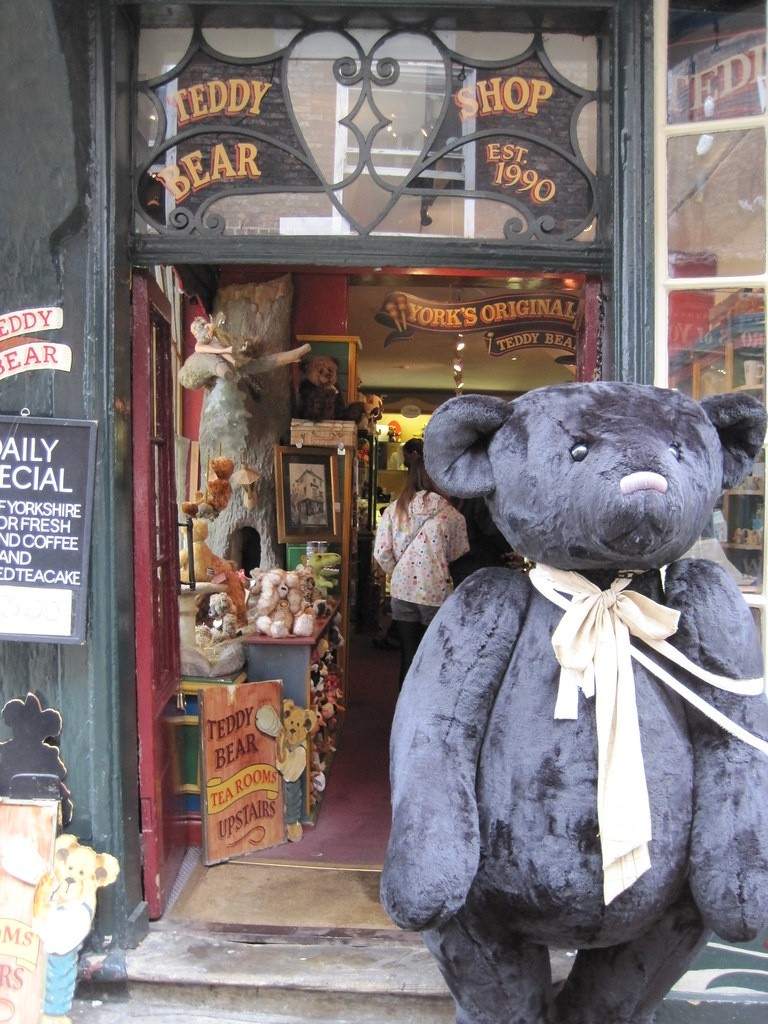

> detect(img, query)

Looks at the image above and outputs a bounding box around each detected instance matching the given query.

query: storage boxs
[380,442,400,470]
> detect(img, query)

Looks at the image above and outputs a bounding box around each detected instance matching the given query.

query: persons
[373,439,505,691]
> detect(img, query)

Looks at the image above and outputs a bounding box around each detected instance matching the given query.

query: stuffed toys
[179,312,402,805]
[380,381,768,1024]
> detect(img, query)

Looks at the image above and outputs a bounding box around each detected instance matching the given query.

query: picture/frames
[273,446,342,543]
[292,335,358,417]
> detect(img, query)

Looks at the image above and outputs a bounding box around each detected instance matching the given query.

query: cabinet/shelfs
[245,595,342,831]
[669,310,765,610]
[179,672,249,848]
[357,430,409,615]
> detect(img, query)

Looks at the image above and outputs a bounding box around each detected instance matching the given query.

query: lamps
[454,333,465,396]
[420,119,439,137]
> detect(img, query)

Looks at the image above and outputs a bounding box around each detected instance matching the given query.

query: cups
[743,360,765,385]
